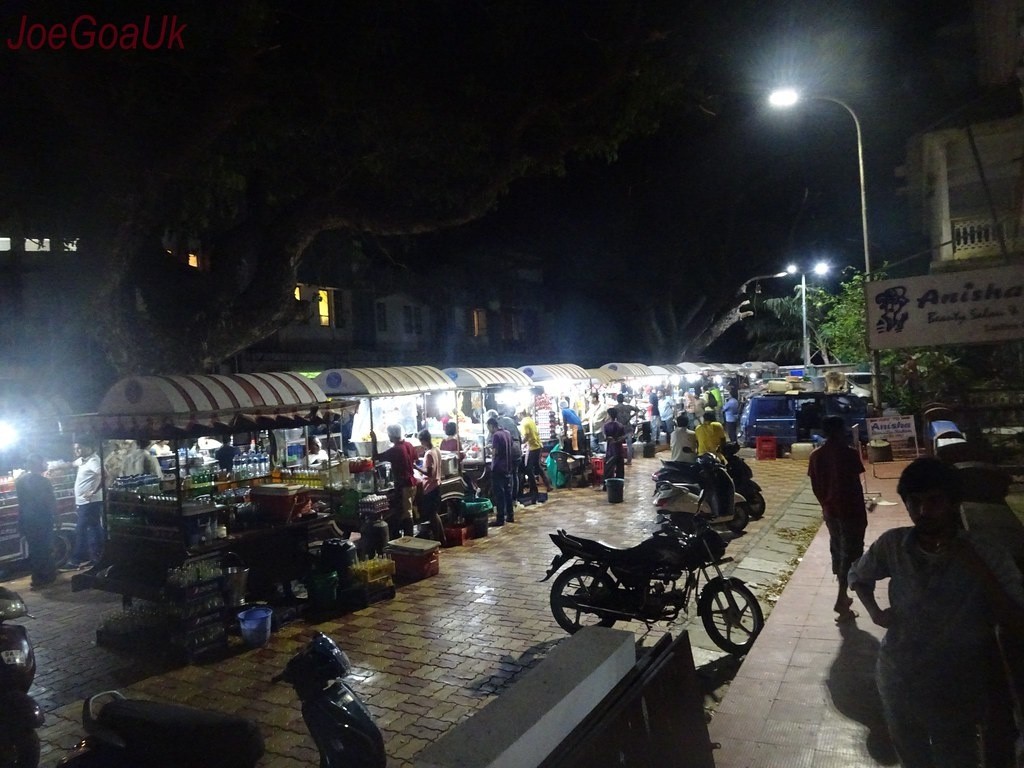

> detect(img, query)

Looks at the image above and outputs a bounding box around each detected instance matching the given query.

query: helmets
[696,453,721,465]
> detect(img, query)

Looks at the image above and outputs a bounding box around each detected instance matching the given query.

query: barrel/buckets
[237,607,273,645]
[605,479,624,503]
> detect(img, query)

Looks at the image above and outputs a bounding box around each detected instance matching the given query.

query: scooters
[652,446,750,537]
[652,431,766,518]
[0,585,45,768]
[55,630,387,768]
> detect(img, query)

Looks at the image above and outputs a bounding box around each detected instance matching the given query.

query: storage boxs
[94,574,229,663]
[180,500,226,543]
[444,522,473,545]
[755,436,776,461]
[391,550,439,580]
[590,457,614,485]
[249,484,311,520]
[346,557,396,593]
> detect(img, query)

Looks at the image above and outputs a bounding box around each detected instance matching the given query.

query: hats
[74,435,94,446]
[514,406,526,416]
[559,400,568,409]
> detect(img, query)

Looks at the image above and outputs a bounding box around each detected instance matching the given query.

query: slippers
[835,610,859,622]
[834,598,853,612]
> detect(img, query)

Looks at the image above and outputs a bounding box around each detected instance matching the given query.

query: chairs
[549,451,587,491]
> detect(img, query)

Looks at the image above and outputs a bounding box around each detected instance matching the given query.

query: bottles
[633,438,643,459]
[102,445,395,656]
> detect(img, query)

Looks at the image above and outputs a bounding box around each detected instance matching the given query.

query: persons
[847,457,1024,767]
[120,440,163,479]
[602,409,626,490]
[721,388,739,441]
[808,415,866,621]
[560,386,748,464]
[15,453,59,586]
[215,435,241,471]
[300,434,328,465]
[46,439,107,571]
[440,422,466,471]
[484,418,515,525]
[669,415,698,463]
[150,440,170,455]
[369,425,419,538]
[486,409,525,492]
[412,430,446,543]
[516,408,553,492]
[695,412,728,466]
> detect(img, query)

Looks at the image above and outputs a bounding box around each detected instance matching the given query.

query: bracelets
[91,491,94,495]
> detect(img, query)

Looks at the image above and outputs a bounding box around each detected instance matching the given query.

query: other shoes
[489,520,504,527]
[506,517,514,523]
[58,562,80,572]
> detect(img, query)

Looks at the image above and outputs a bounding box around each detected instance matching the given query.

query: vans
[737,393,870,447]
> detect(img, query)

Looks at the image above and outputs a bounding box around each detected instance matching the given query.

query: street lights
[770,89,882,416]
[788,262,829,366]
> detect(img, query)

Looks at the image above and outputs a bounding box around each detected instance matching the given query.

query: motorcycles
[535,489,764,656]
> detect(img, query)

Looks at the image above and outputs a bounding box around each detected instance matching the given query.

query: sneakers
[83,560,98,569]
